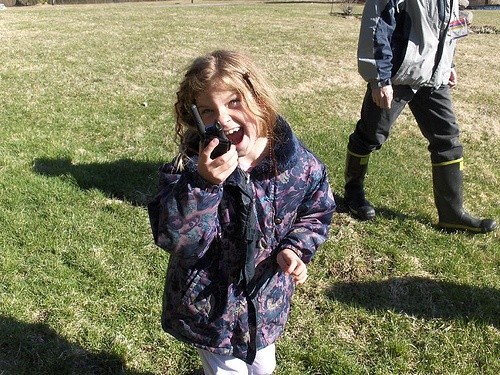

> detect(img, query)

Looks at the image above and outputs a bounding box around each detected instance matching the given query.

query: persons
[343,0,495,232]
[147,51,338,375]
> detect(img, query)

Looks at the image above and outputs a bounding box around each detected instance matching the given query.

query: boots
[345,146,375,221]
[431,157,497,231]
